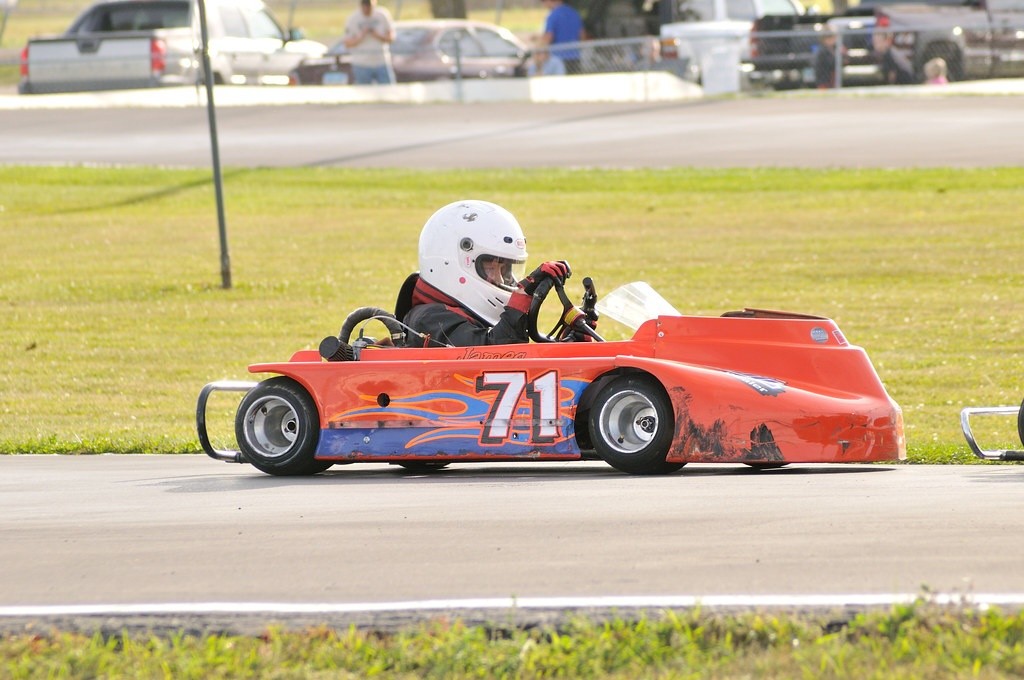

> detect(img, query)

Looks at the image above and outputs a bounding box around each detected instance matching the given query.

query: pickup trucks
[18,0,331,95]
[748,0,1024,90]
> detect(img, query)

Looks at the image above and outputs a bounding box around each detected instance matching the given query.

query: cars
[564,0,822,85]
[289,18,527,85]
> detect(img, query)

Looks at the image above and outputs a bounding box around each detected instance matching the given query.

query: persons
[344,0,396,83]
[537,0,588,73]
[634,38,673,72]
[923,58,949,83]
[872,33,914,83]
[406,199,599,348]
[815,28,848,88]
[527,51,568,78]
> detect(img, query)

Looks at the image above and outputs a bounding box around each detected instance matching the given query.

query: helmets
[419,199,527,327]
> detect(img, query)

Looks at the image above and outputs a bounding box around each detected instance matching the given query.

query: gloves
[518,259,572,296]
[557,314,596,342]
